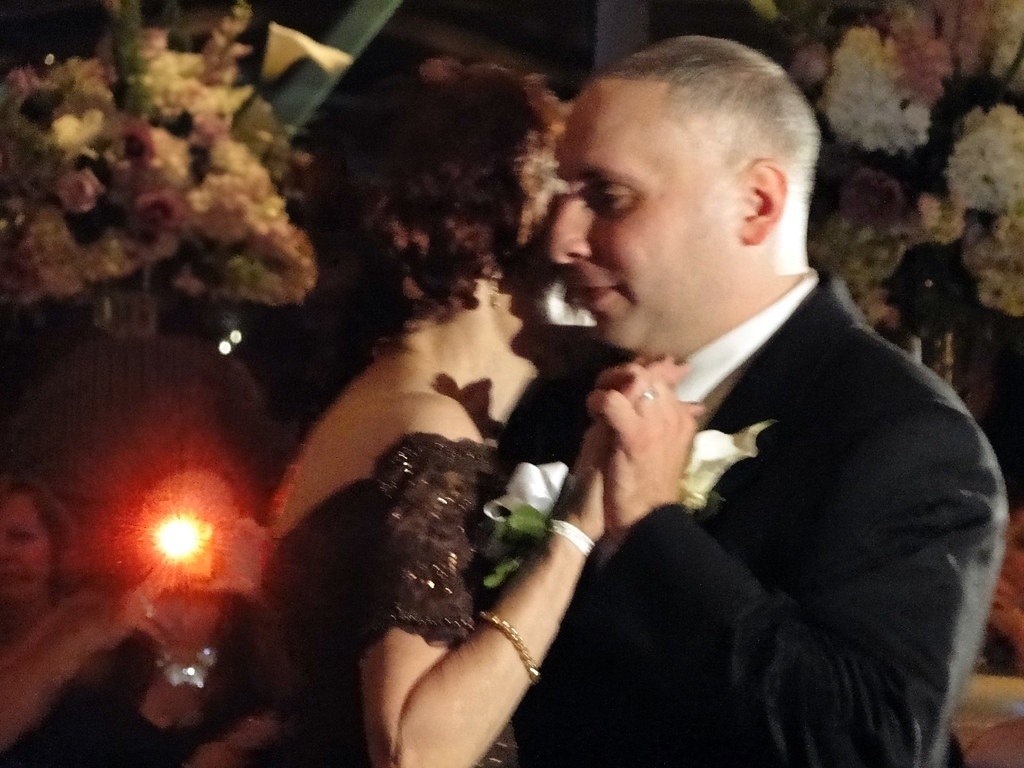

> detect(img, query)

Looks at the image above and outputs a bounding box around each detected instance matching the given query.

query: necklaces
[149,638,220,690]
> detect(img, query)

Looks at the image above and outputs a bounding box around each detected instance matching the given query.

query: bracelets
[243,715,282,747]
[547,518,596,558]
[478,610,543,684]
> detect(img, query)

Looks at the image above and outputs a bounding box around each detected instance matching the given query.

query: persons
[0,420,290,768]
[987,507,1024,679]
[461,34,1011,768]
[253,57,707,768]
[0,472,80,654]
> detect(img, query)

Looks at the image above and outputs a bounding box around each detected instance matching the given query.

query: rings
[632,389,657,414]
